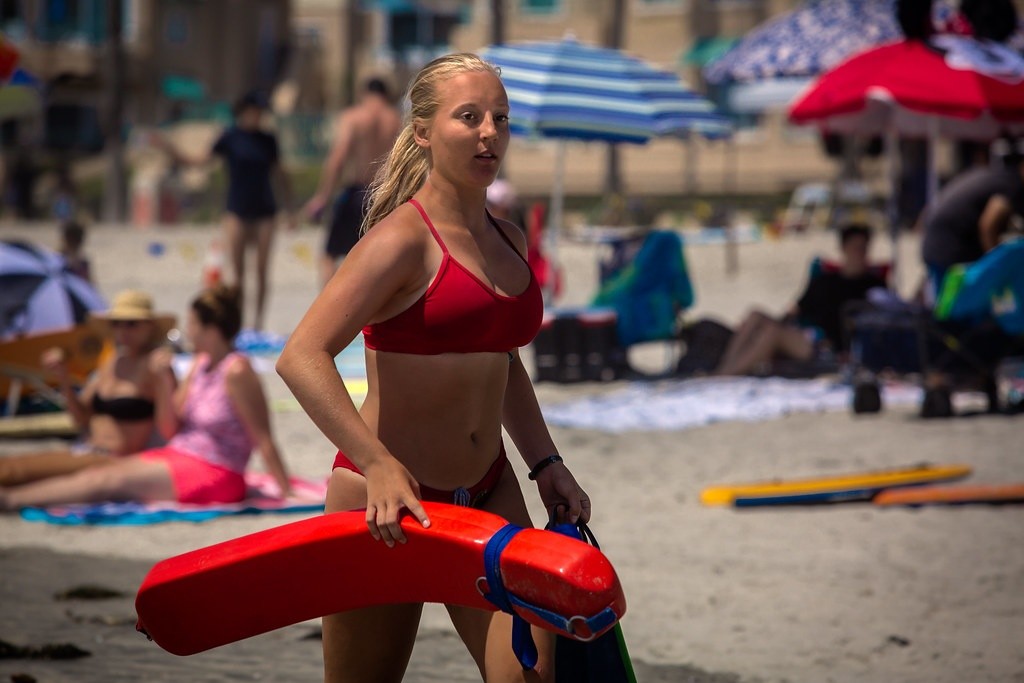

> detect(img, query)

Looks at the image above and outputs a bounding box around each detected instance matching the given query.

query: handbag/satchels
[548,512,640,683]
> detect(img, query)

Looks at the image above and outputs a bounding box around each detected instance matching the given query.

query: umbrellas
[0,236,108,346]
[683,0,1024,227]
[464,39,737,309]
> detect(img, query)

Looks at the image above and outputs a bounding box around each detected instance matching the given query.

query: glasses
[111,321,138,328]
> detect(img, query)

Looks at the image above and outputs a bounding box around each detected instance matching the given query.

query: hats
[86,289,174,348]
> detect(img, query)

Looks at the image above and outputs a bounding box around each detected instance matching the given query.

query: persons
[60,222,91,282]
[150,87,314,332]
[295,57,402,287]
[715,185,1024,418]
[273,51,592,683]
[0,286,292,515]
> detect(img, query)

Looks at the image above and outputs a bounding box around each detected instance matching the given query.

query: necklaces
[203,345,240,372]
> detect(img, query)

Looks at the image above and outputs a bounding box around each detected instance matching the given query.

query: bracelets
[528,454,564,481]
